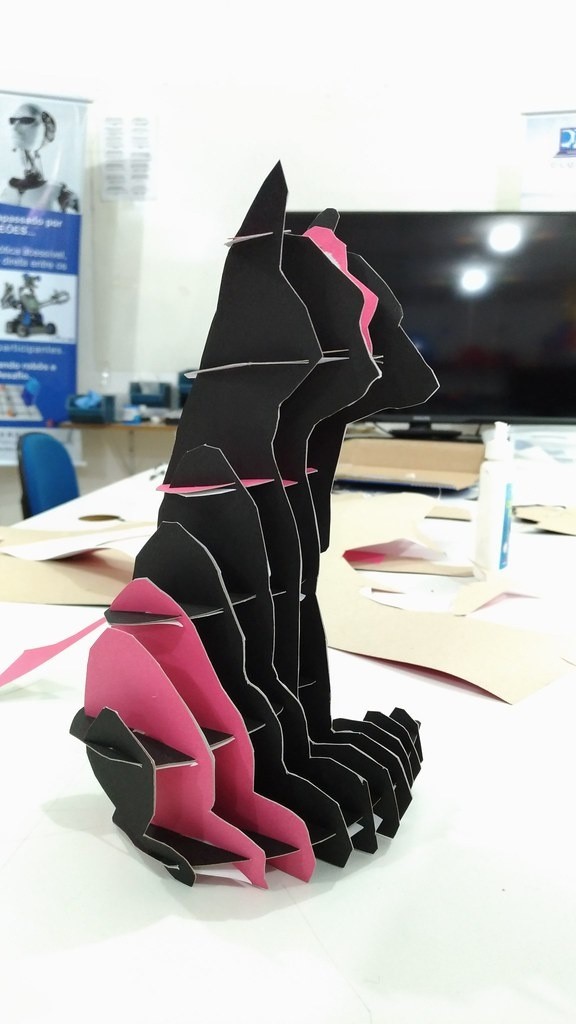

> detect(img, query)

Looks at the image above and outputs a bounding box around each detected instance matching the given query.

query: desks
[59,418,178,475]
[344,432,484,443]
[0,463,575,1024]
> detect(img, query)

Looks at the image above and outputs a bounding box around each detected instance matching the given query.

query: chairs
[17,433,81,520]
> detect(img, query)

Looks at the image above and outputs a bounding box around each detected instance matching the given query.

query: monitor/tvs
[284,211,576,443]
